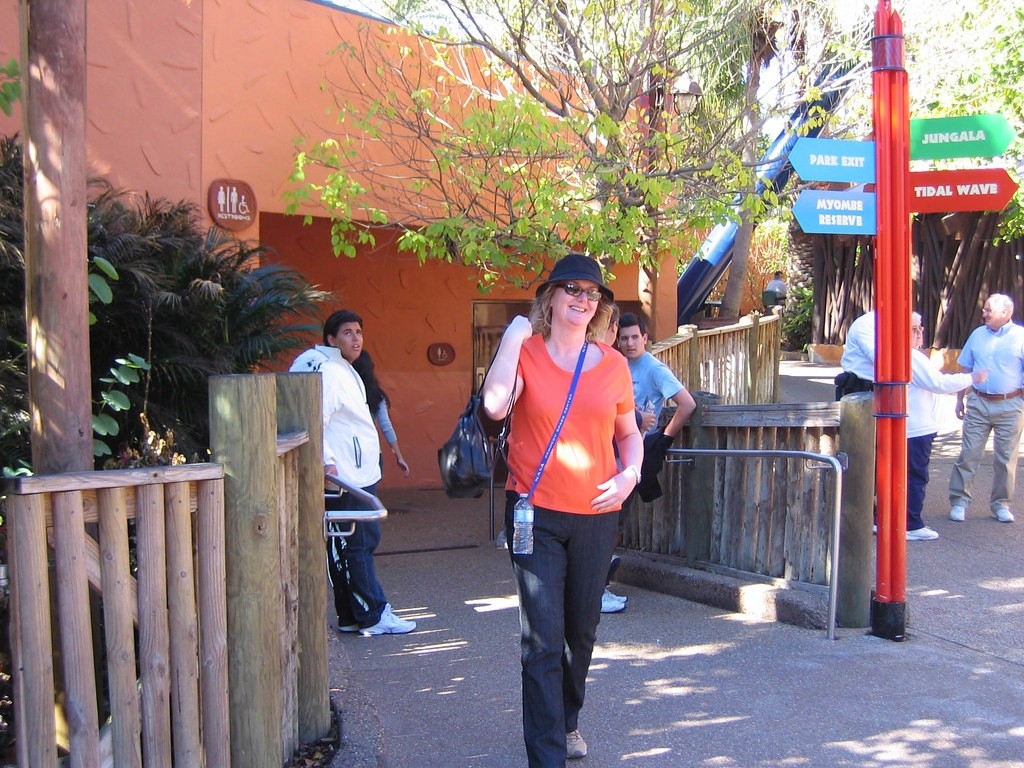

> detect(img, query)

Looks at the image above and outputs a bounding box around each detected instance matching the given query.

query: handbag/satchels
[437,392,513,500]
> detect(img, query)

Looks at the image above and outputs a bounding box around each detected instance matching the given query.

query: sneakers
[601,587,628,613]
[564,730,588,759]
[338,603,417,636]
[906,527,939,541]
[949,505,966,521]
[991,509,1014,522]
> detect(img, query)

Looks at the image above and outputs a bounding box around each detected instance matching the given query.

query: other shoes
[609,555,621,583]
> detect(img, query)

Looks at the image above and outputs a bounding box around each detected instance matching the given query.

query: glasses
[912,326,924,332]
[553,284,602,302]
[981,308,996,314]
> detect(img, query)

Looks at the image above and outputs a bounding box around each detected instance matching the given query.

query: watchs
[631,465,641,484]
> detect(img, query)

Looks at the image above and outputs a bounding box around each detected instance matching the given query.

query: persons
[949,294,1024,522]
[351,349,409,476]
[767,270,787,305]
[289,310,416,636]
[872,311,988,542]
[483,254,644,768]
[601,303,656,612]
[606,312,696,586]
[834,311,874,431]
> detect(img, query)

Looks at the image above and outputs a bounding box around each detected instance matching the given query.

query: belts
[972,386,1023,400]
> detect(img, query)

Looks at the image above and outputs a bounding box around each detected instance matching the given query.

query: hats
[535,255,615,306]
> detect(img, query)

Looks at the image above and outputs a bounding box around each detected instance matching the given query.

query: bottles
[513,492,534,554]
